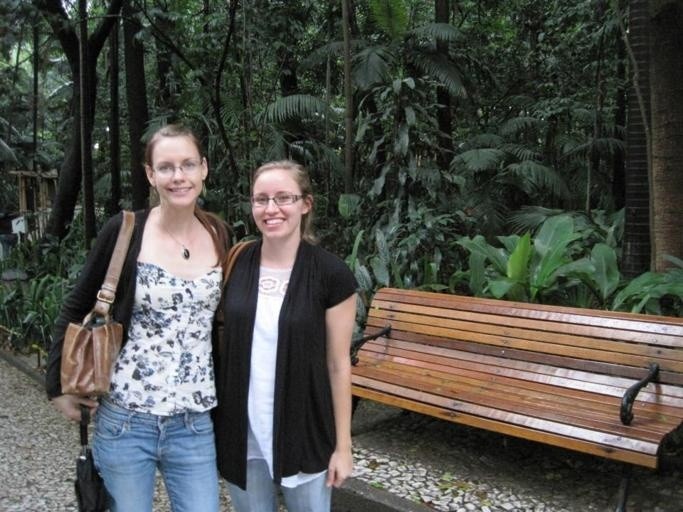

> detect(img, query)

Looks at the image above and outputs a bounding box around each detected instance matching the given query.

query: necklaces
[159,213,196,260]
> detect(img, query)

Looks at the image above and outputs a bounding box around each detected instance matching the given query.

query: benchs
[349,287,683,511]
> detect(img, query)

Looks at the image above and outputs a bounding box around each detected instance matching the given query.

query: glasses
[250,194,306,207]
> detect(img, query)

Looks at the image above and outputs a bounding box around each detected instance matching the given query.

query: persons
[44,123,234,512]
[210,158,360,512]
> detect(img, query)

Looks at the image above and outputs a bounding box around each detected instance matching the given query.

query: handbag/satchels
[60,318,124,396]
